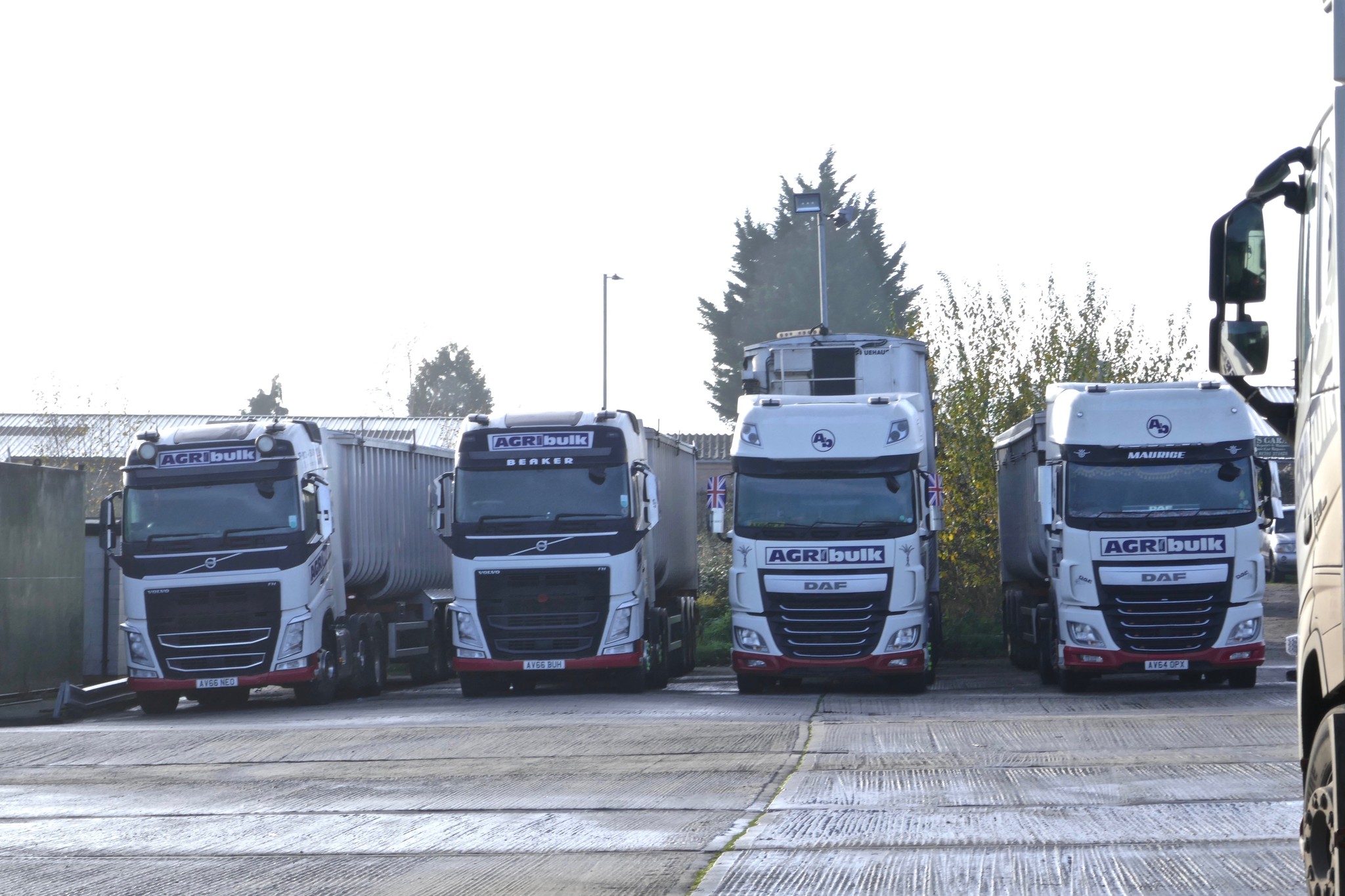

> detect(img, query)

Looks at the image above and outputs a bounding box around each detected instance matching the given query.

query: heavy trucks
[1210,0,1345,896]
[99,330,1301,715]
